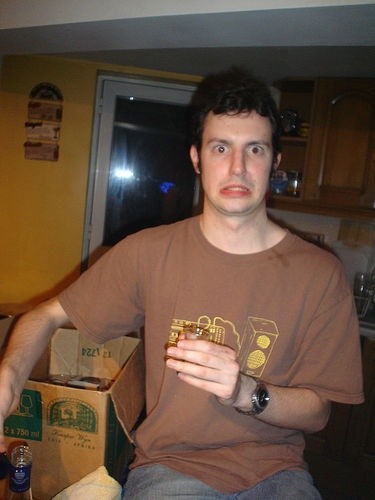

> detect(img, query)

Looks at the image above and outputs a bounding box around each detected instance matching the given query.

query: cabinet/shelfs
[304,336,375,472]
[266,76,375,220]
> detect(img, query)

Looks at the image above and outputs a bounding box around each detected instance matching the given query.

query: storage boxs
[0,315,145,500]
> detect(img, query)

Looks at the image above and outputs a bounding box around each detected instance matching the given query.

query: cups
[287,177,301,197]
[271,171,287,194]
[184,328,209,340]
[354,272,369,297]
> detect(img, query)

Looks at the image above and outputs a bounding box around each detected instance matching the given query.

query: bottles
[6,445,32,500]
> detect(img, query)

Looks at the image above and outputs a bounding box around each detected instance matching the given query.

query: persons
[0,73,364,500]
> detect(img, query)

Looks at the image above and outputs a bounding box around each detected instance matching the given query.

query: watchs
[236,377,270,415]
